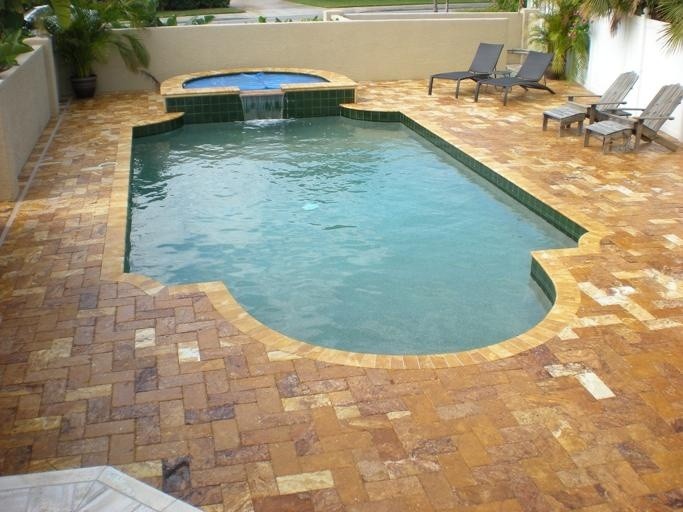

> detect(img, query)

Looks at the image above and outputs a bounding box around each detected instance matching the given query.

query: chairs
[427,41,683,149]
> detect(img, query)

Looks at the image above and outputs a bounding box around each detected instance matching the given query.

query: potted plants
[27,1,151,98]
